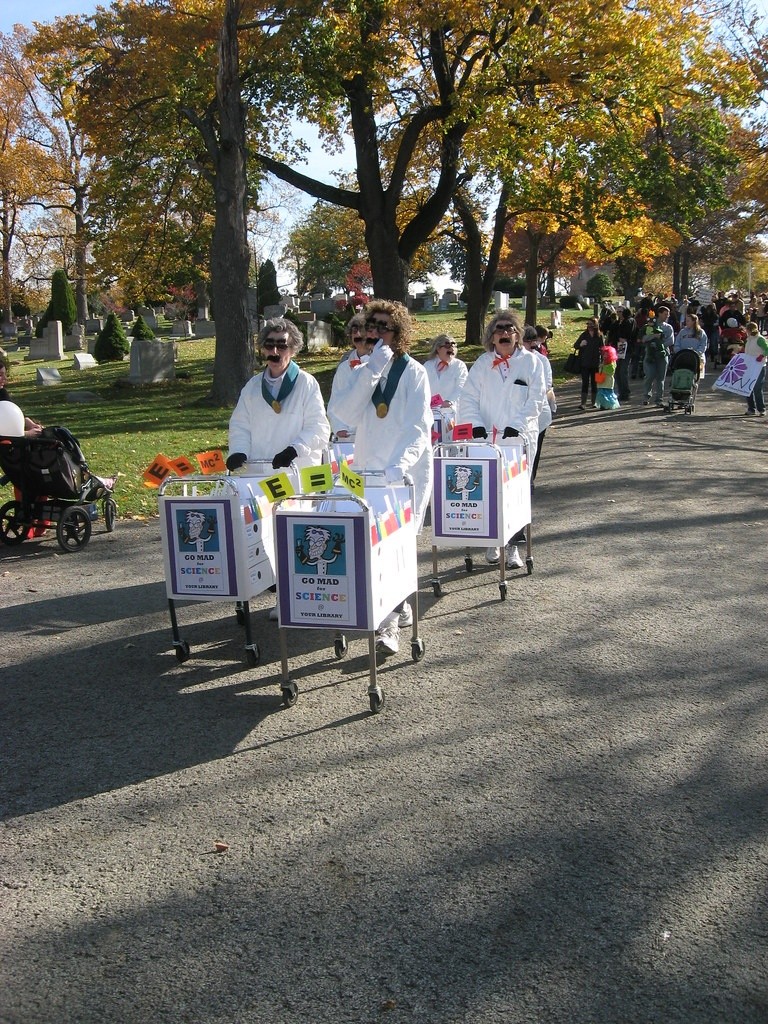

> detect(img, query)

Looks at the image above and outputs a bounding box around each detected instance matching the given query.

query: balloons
[0,400,25,436]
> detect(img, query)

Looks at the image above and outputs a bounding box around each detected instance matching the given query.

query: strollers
[663,346,702,416]
[0,425,118,552]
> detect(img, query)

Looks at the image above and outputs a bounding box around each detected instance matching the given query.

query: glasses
[365,323,396,333]
[261,340,291,351]
[493,326,518,335]
[352,326,364,334]
[440,343,457,348]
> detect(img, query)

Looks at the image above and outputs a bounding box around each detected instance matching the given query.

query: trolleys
[429,430,534,601]
[158,458,301,668]
[270,469,427,714]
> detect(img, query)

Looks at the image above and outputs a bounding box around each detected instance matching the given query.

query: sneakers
[375,619,401,654]
[485,547,500,565]
[505,545,524,568]
[399,604,413,626]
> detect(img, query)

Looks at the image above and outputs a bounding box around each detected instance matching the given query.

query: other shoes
[578,404,586,410]
[269,607,278,620]
[745,411,755,415]
[657,400,664,408]
[593,403,597,408]
[642,396,650,405]
[760,410,766,417]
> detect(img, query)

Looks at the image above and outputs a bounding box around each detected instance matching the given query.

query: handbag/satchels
[594,367,606,383]
[565,349,581,375]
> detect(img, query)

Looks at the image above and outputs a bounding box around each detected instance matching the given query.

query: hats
[727,318,738,327]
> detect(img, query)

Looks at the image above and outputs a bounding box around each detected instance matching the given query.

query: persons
[14,486,51,539]
[599,291,768,380]
[226,318,331,619]
[642,306,675,407]
[745,322,768,416]
[639,310,666,363]
[460,313,547,568]
[574,318,604,409]
[326,300,434,654]
[595,346,620,410]
[0,360,44,436]
[423,334,469,408]
[674,314,709,364]
[615,309,639,401]
[523,324,553,479]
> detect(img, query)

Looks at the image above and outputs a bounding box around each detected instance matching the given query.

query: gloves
[473,427,488,439]
[501,427,519,439]
[384,464,404,484]
[367,338,394,374]
[226,453,247,472]
[756,355,764,362]
[272,446,298,469]
